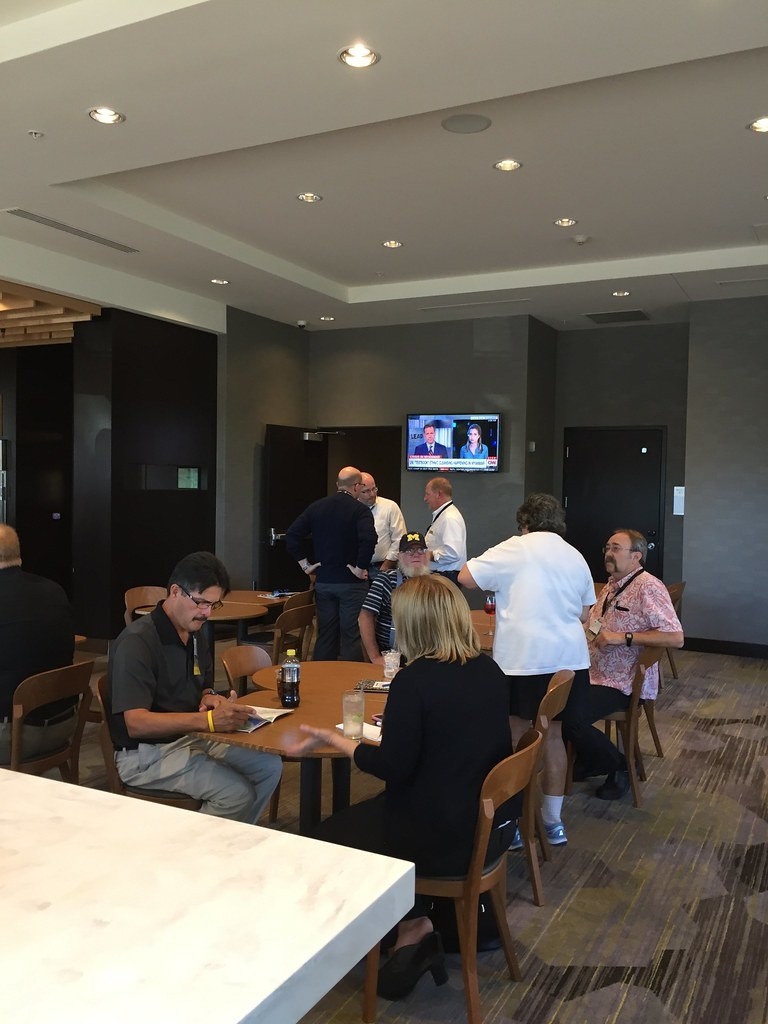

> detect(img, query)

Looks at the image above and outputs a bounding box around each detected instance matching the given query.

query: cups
[343,690,364,739]
[274,669,281,698]
[381,650,401,678]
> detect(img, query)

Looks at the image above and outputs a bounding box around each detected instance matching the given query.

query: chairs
[0,580,682,1024]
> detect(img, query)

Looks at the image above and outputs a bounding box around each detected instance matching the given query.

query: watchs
[302,563,310,570]
[623,632,633,647]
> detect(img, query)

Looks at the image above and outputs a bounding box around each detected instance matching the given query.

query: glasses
[359,487,378,496]
[400,546,425,556]
[601,546,638,555]
[517,522,524,533]
[168,583,224,612]
[352,484,366,489]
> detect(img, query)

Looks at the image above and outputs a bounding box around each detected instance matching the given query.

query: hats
[399,532,427,549]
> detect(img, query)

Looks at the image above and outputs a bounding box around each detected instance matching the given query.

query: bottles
[281,649,301,709]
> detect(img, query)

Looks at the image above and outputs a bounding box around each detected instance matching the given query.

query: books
[234,707,295,733]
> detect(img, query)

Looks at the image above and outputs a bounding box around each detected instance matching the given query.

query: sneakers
[596,764,640,800]
[543,821,568,845]
[571,759,604,781]
[507,828,522,851]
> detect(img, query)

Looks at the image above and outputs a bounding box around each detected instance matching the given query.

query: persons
[0,521,78,764]
[284,467,466,663]
[460,424,488,459]
[553,529,684,799]
[108,552,283,824]
[455,494,598,849]
[312,574,519,1001]
[414,424,448,458]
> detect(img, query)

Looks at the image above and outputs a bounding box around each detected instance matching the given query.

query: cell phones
[372,713,383,723]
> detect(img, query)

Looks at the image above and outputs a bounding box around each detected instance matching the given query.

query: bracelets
[206,710,215,733]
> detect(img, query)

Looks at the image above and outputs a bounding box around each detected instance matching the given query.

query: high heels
[376,930,448,999]
[423,901,458,952]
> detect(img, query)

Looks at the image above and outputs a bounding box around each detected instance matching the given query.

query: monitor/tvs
[406,413,501,473]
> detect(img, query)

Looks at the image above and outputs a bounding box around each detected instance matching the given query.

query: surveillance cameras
[298,320,306,328]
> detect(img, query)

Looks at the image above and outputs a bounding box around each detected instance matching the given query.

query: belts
[0,705,80,728]
[112,744,139,752]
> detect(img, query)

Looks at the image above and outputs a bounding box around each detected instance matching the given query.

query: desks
[219,589,293,620]
[251,660,398,813]
[135,602,267,684]
[190,689,388,834]
[465,608,497,652]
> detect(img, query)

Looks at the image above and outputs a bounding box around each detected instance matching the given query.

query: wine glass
[483,595,496,636]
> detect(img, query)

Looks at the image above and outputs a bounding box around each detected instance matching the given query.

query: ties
[428,445,434,456]
